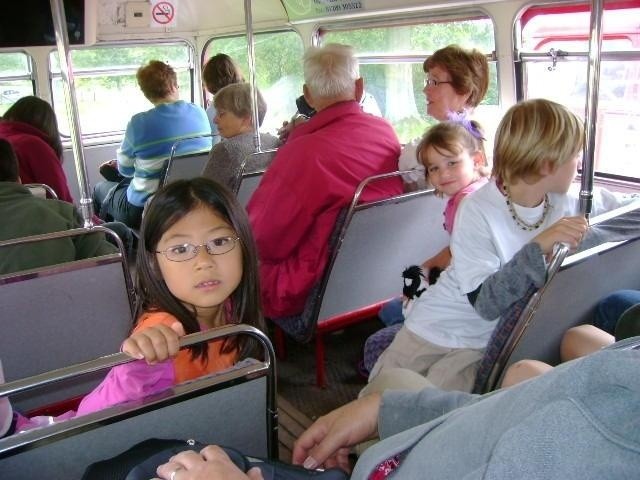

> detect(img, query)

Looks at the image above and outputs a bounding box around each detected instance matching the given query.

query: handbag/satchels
[126,444,349,480]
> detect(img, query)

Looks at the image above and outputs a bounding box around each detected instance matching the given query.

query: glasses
[155,236,240,262]
[424,79,454,88]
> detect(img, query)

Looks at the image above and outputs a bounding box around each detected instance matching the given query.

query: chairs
[2,322,278,479]
[24,182,59,203]
[267,169,450,390]
[349,335,640,480]
[234,148,279,212]
[1,226,137,412]
[361,198,640,394]
[162,131,222,186]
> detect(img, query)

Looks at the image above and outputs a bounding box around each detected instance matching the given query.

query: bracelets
[503,183,549,231]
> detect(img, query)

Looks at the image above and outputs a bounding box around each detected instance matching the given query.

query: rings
[170,467,182,480]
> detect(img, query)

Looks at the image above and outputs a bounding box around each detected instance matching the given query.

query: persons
[1,319,188,440]
[133,176,260,384]
[203,53,245,149]
[0,95,74,205]
[91,60,212,230]
[81,334,640,480]
[398,45,488,190]
[0,138,119,276]
[502,322,615,387]
[199,83,283,192]
[366,99,589,392]
[593,289,640,338]
[246,45,406,318]
[359,120,488,379]
